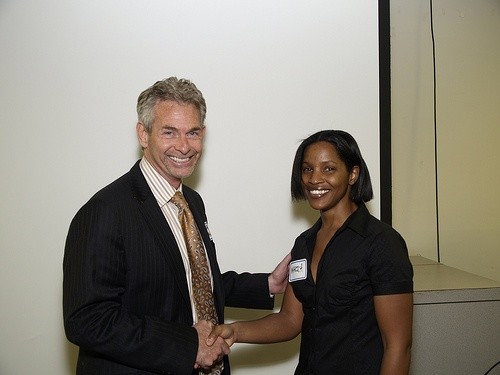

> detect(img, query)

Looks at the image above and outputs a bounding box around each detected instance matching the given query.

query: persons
[194,129,415,375]
[61,76,292,375]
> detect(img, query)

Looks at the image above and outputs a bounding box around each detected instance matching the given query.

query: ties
[168,192,225,374]
